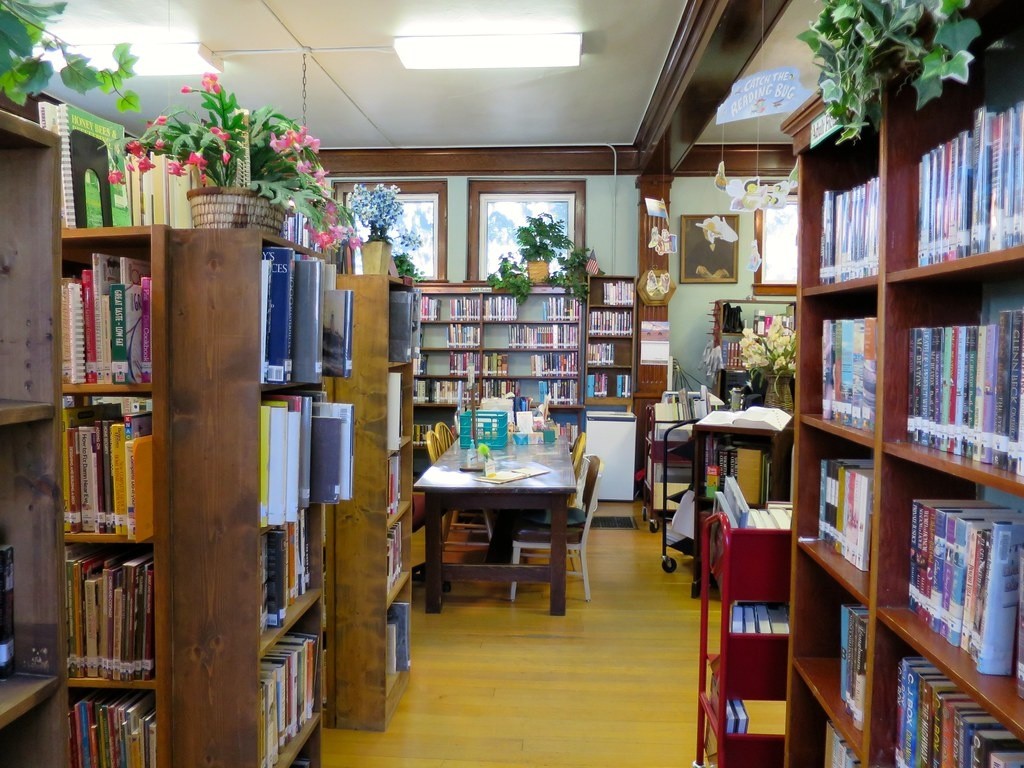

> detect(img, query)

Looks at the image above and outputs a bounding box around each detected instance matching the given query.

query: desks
[413,426,577,616]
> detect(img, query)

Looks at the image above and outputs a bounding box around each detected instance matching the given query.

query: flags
[586,248,598,275]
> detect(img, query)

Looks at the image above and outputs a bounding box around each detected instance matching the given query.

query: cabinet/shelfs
[0,0,1024,768]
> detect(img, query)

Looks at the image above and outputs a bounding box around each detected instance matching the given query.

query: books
[818,457,874,571]
[725,698,750,733]
[840,602,869,731]
[825,720,862,768]
[820,176,881,284]
[587,281,634,398]
[906,310,1024,477]
[713,476,794,530]
[38,100,422,768]
[822,318,878,434]
[722,303,797,366]
[0,545,16,679]
[895,657,1024,768]
[730,604,790,635]
[413,296,578,447]
[918,100,1024,266]
[908,499,1024,700]
[655,385,712,441]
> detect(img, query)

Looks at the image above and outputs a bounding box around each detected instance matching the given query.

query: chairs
[511,431,586,568]
[434,420,496,529]
[508,454,604,604]
[425,429,493,551]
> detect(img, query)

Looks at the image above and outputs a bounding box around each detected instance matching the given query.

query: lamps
[20,41,224,75]
[392,33,583,70]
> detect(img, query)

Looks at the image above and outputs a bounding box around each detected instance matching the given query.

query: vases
[186,188,288,238]
[359,240,395,275]
[763,374,794,414]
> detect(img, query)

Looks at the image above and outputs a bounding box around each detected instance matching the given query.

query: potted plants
[485,211,591,305]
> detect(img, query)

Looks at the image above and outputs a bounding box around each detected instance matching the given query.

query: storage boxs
[459,410,508,451]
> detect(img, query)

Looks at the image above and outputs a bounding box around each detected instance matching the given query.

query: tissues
[509,411,545,445]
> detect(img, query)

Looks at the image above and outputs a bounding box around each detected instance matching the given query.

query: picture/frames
[680,213,739,284]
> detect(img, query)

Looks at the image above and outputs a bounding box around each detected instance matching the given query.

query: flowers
[391,225,429,277]
[732,316,796,397]
[345,182,406,243]
[94,71,360,253]
